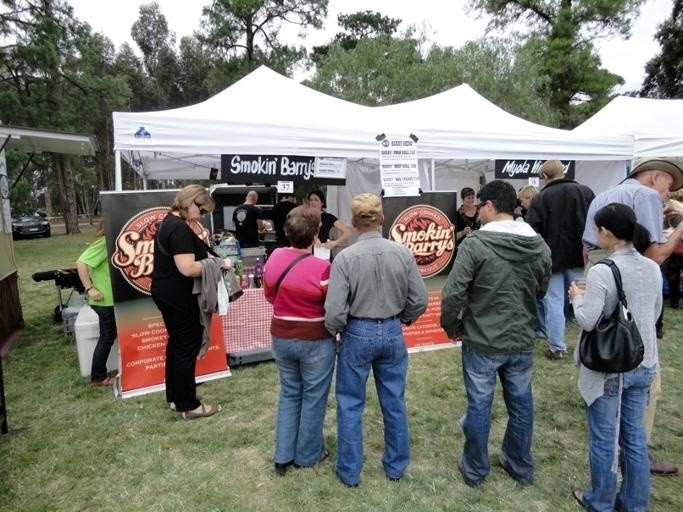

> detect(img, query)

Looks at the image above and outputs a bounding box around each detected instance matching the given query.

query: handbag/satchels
[223,268,244,301]
[579,260,644,373]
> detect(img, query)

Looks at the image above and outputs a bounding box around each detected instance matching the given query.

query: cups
[570,278,585,303]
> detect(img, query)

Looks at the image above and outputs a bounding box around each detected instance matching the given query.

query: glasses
[475,199,496,209]
[195,200,208,215]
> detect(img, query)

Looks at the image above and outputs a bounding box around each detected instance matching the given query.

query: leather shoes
[170,395,202,411]
[649,457,678,474]
[182,403,222,420]
[573,488,585,503]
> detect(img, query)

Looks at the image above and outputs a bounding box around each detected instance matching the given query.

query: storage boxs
[75,305,119,376]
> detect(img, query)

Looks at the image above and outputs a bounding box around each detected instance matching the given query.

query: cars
[12,212,51,237]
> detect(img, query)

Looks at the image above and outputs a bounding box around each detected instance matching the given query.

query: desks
[218,283,341,367]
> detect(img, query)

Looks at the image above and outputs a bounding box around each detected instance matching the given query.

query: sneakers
[543,349,562,360]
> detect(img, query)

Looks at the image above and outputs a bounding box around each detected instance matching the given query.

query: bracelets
[84,285,96,293]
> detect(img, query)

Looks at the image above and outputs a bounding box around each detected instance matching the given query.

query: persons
[232,189,354,263]
[149,184,234,420]
[452,161,596,359]
[262,205,337,475]
[323,192,428,488]
[440,180,553,488]
[76,234,115,386]
[581,157,683,475]
[566,203,664,512]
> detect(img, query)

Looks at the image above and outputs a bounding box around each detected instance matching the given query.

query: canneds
[235,260,255,289]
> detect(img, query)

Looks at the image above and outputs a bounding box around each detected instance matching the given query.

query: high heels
[276,459,294,475]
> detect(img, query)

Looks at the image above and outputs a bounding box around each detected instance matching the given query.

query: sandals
[95,376,115,387]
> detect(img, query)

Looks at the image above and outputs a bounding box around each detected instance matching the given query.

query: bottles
[255,258,263,288]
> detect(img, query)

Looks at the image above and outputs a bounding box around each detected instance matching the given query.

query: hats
[351,192,382,223]
[618,154,682,191]
